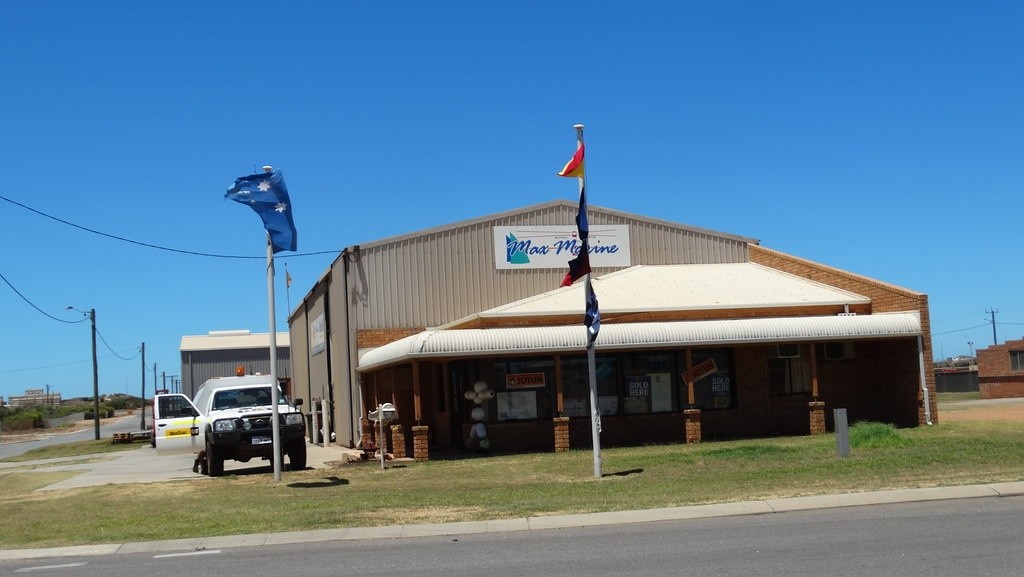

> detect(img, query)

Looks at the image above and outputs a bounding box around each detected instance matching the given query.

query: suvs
[153,365,307,477]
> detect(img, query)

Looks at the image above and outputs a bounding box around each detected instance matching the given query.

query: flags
[224,168,297,254]
[558,132,600,351]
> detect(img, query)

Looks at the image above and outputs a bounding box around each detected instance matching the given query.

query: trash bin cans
[304,411,323,444]
